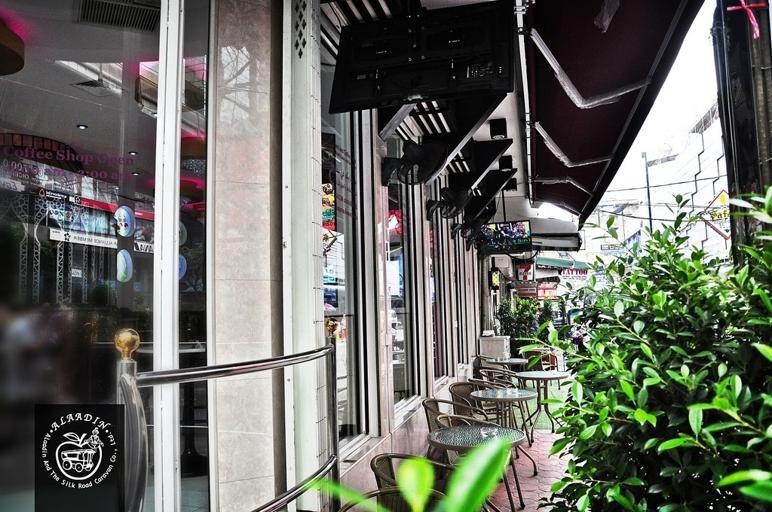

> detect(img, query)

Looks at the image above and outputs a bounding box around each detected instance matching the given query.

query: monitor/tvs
[481,220,533,254]
[329,0,516,114]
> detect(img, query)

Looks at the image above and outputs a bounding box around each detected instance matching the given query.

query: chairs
[420,351,570,459]
[336,486,446,511]
[369,451,501,512]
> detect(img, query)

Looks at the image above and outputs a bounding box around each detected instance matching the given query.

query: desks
[426,424,527,512]
[469,387,540,485]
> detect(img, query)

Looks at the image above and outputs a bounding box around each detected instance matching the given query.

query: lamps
[499,155,513,172]
[488,118,508,141]
[488,266,501,293]
[505,177,518,193]
